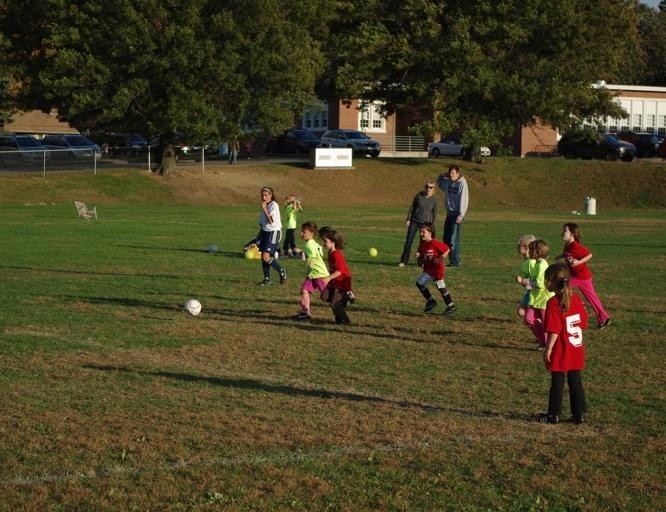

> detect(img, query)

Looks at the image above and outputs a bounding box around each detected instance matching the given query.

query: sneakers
[424,300,437,312]
[293,312,310,321]
[258,278,272,285]
[347,291,354,304]
[280,268,286,284]
[569,415,586,424]
[597,318,612,330]
[537,413,560,424]
[444,305,457,315]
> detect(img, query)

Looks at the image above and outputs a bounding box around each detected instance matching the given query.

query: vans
[103,134,148,158]
[0,134,52,167]
[41,134,100,164]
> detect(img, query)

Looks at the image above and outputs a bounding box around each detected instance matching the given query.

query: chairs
[74,200,97,221]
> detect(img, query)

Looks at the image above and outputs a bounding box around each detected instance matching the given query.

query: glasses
[425,185,435,190]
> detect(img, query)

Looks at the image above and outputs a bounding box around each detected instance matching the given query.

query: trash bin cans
[582,197,596,215]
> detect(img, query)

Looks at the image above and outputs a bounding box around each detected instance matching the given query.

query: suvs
[320,130,381,159]
[556,127,636,164]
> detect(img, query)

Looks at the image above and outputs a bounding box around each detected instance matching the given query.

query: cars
[617,130,665,158]
[174,141,208,162]
[427,136,491,159]
[86,135,113,161]
[265,129,321,153]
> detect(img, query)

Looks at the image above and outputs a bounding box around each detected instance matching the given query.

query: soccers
[369,247,378,256]
[208,244,217,253]
[184,300,202,316]
[245,249,255,259]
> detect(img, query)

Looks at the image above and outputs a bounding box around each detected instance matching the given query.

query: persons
[537,263,588,423]
[259,186,286,286]
[415,222,457,315]
[515,223,612,351]
[398,180,438,266]
[291,221,355,325]
[437,165,469,267]
[228,137,239,166]
[283,195,303,256]
